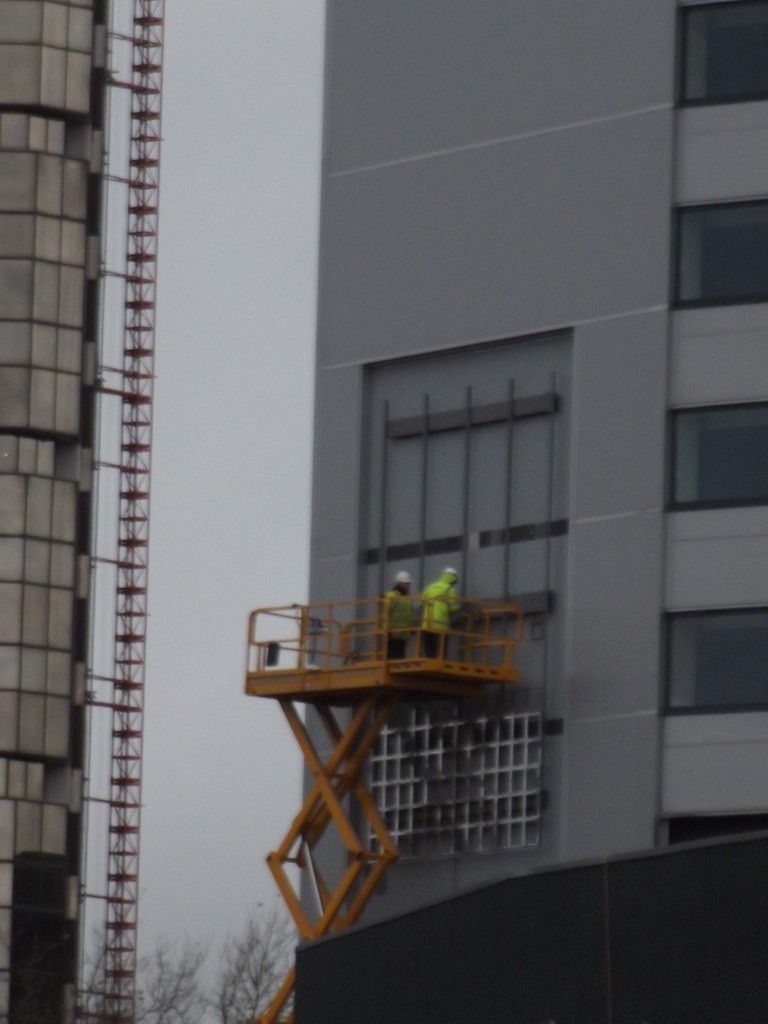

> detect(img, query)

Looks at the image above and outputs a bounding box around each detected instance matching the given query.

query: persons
[419,567,460,660]
[382,571,416,661]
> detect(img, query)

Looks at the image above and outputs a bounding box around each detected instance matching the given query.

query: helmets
[445,568,457,576]
[395,571,413,584]
